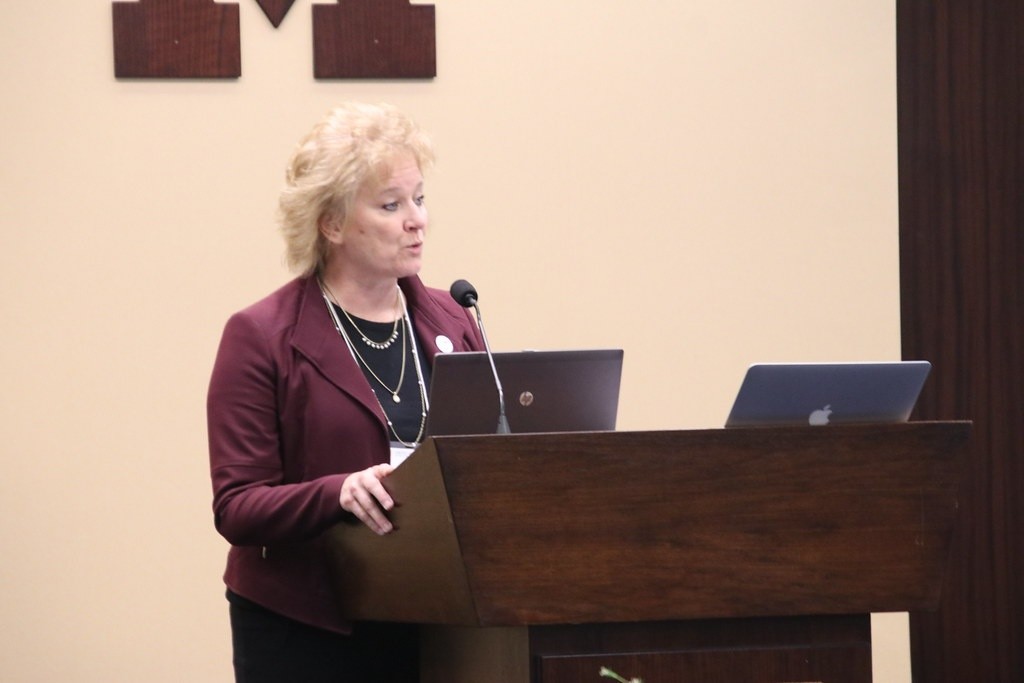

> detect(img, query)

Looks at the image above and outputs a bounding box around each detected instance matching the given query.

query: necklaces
[317,275,429,447]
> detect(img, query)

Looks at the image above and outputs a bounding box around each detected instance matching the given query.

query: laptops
[725,360,932,426]
[424,349,624,439]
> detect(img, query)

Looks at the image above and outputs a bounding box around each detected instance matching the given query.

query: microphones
[450,278,512,435]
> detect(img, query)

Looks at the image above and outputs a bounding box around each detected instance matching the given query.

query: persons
[207,102,486,682]
